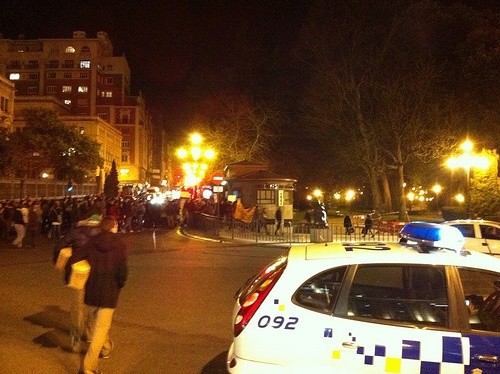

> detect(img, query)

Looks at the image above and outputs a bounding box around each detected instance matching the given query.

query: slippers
[80,369,101,374]
[100,339,115,358]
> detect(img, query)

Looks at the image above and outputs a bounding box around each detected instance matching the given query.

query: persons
[54,208,129,374]
[486,227,500,240]
[343,215,352,235]
[0,192,313,248]
[362,215,374,238]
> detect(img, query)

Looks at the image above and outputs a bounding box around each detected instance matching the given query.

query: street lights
[445,140,489,187]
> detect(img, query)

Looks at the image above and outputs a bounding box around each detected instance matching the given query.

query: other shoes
[82,331,89,341]
[73,348,86,353]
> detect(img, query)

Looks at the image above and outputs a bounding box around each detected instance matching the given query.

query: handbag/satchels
[362,228,367,234]
[350,228,355,232]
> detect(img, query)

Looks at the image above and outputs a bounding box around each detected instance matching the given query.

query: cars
[225,221,500,374]
[440,220,500,258]
